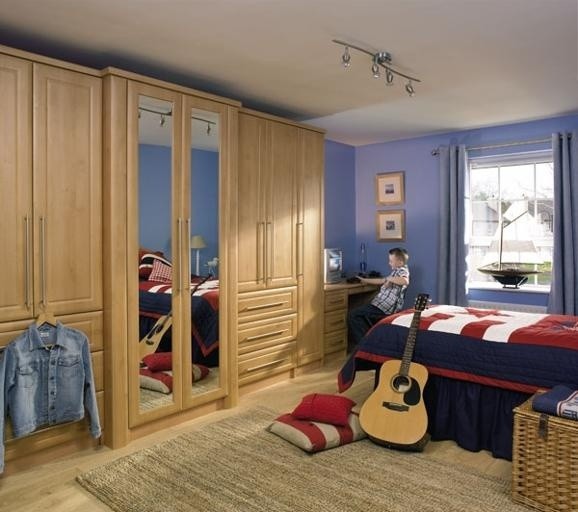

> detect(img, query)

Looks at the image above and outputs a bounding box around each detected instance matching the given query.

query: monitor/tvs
[324,247,343,283]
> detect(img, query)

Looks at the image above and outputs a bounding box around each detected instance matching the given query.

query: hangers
[32,298,59,330]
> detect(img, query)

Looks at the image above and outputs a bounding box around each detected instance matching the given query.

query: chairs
[360,275,411,331]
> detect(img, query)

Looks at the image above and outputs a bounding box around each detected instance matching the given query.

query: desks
[324,282,381,368]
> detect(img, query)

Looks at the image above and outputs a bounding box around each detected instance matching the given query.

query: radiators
[468,299,548,315]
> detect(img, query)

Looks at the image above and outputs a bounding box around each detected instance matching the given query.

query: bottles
[359,243,367,272]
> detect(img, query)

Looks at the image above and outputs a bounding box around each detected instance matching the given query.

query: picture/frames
[376,171,406,204]
[378,210,405,243]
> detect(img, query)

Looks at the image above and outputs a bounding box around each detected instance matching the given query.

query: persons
[349,247,410,350]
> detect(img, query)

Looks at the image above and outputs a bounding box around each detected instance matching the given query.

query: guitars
[139,271,213,368]
[360,293,432,451]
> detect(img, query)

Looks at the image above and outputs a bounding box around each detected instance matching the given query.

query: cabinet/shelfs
[103,66,238,450]
[238,108,325,405]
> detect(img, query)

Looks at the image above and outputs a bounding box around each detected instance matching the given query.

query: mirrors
[138,92,173,410]
[191,108,220,399]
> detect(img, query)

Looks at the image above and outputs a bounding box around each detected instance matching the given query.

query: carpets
[75,405,528,512]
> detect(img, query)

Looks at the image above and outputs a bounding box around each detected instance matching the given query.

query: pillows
[265,394,366,454]
[139,353,209,394]
[138,250,173,283]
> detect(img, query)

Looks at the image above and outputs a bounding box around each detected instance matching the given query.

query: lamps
[332,39,420,97]
[191,236,206,276]
[139,106,173,125]
[191,116,216,135]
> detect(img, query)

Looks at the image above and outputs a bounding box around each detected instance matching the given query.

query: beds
[138,276,220,364]
[337,301,578,462]
